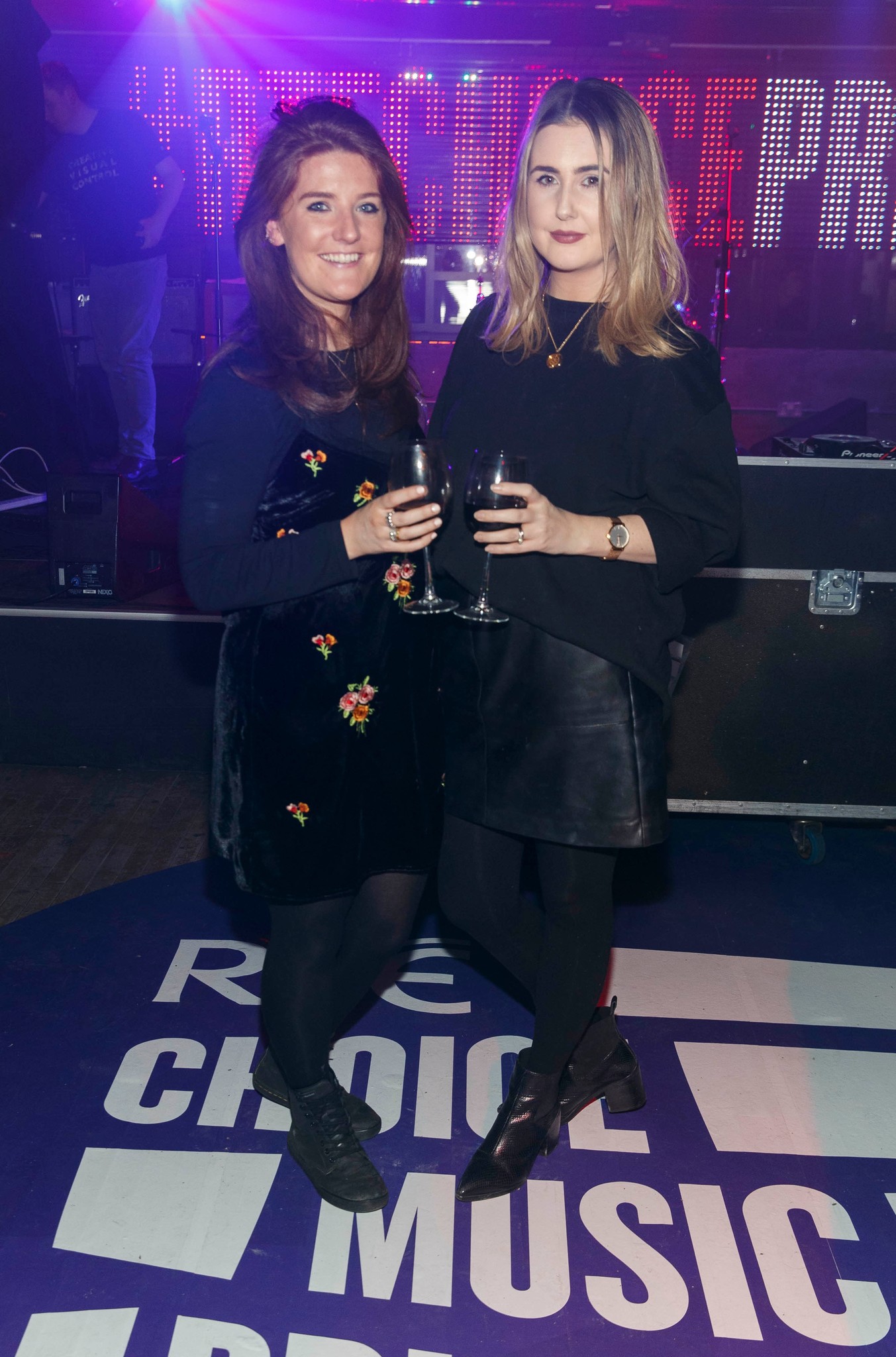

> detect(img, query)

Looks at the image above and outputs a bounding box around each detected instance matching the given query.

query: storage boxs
[664,455,895,818]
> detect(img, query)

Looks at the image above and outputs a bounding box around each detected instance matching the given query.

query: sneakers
[253,1040,382,1141]
[288,1080,385,1212]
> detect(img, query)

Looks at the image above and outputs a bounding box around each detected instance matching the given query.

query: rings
[385,511,401,530]
[517,526,525,544]
[389,528,399,542]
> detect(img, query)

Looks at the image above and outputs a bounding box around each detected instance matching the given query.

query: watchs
[604,515,630,561]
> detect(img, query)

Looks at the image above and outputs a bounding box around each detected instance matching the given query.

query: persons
[37,60,189,602]
[174,95,436,1213]
[435,76,738,1203]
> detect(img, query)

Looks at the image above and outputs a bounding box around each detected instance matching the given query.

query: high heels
[558,995,643,1123]
[454,1050,562,1199]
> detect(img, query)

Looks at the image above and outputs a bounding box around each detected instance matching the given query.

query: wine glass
[451,448,527,623]
[386,438,459,616]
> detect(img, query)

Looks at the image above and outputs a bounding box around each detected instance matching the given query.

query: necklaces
[541,276,601,370]
[304,330,362,414]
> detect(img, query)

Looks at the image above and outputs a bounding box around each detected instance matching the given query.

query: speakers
[44,471,155,603]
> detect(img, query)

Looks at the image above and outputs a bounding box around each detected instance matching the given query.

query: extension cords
[0,491,47,513]
